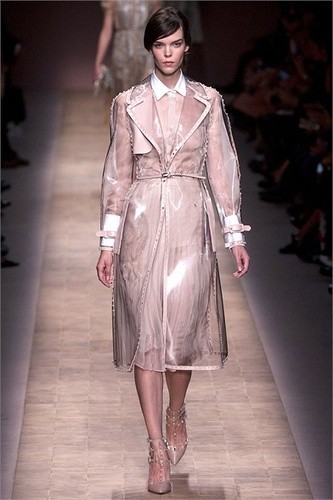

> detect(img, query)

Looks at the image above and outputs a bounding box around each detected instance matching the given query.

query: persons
[96,9,252,495]
[92,0,166,97]
[213,0,333,297]
[0,27,29,268]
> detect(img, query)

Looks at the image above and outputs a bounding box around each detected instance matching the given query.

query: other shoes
[1,260,19,268]
[319,255,332,267]
[328,284,332,294]
[145,438,172,493]
[242,129,308,243]
[320,265,332,276]
[1,236,5,242]
[1,158,29,168]
[1,250,10,256]
[166,399,188,465]
[1,199,11,208]
[279,244,295,254]
[1,182,10,192]
